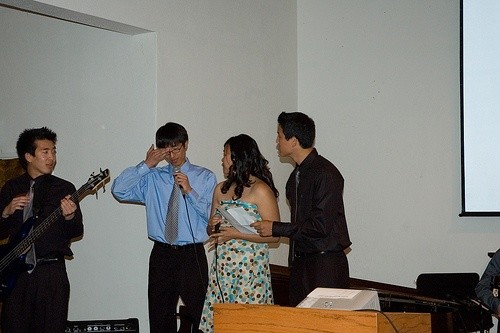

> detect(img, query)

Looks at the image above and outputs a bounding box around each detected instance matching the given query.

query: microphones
[173,167,185,200]
[214,213,222,249]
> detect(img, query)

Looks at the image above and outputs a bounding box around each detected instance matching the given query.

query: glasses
[164,144,183,156]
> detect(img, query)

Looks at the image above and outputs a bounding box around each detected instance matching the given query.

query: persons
[0,125,85,333]
[199,135,281,333]
[250,112,352,306]
[111,122,218,333]
[474,250,500,315]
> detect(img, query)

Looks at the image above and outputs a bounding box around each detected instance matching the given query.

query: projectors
[297,287,381,312]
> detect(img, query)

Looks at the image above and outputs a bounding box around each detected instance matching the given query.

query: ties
[164,171,181,246]
[22,180,37,275]
[287,167,301,269]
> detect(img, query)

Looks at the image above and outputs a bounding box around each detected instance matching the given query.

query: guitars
[0,167,111,303]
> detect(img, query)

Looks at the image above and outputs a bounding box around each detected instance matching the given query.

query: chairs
[416,273,494,333]
